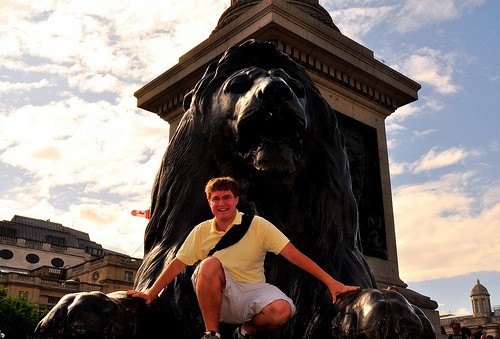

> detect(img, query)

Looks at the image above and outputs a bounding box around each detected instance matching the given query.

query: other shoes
[233,326,246,339]
[201,331,220,339]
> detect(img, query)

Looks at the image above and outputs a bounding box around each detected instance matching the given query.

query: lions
[34,39,436,339]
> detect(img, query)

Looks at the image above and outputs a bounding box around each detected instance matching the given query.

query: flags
[131,209,150,219]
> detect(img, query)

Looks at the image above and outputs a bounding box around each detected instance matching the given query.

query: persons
[448,323,494,339]
[127,176,361,339]
[390,286,399,292]
